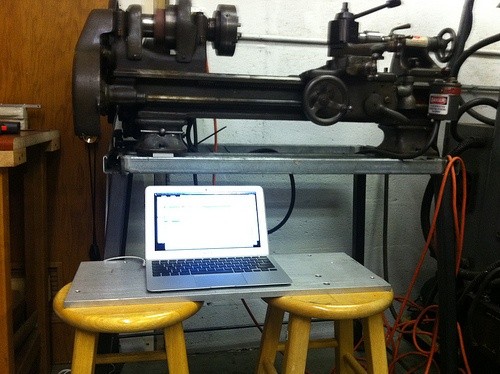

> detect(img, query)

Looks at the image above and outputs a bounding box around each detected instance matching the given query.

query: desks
[0,129,61,374]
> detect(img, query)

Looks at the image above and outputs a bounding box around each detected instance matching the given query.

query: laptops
[145,185,293,293]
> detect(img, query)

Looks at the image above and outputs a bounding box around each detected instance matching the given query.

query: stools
[52,283,204,374]
[255,288,392,374]
